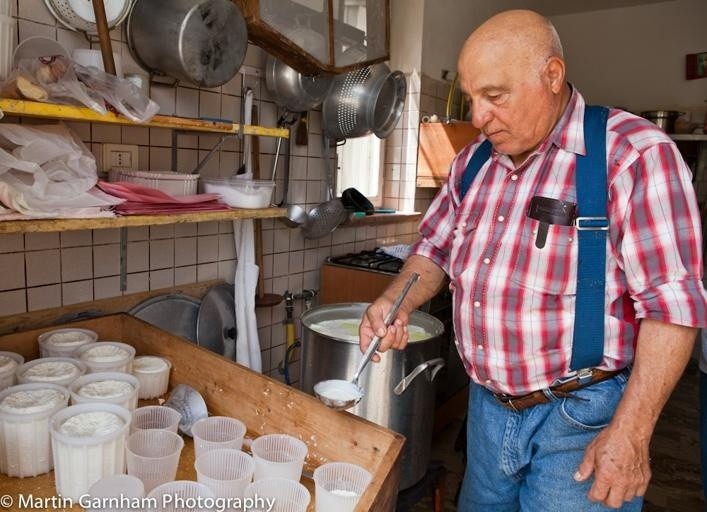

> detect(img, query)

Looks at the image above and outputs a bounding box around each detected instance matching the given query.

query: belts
[495,366,625,412]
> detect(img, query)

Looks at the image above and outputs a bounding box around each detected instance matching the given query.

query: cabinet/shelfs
[415,120,482,191]
[0,96,291,235]
[316,256,470,510]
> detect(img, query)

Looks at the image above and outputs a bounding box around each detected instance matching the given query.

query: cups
[123,73,149,102]
[0,329,374,512]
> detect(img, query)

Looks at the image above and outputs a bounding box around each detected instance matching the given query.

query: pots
[125,0,250,90]
[265,16,333,123]
[639,110,686,132]
[325,37,410,151]
[300,302,449,489]
[675,121,705,134]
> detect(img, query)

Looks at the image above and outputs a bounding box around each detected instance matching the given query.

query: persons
[359,9,707,512]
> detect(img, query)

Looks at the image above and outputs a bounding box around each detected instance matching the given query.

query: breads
[0,76,49,101]
[40,62,67,81]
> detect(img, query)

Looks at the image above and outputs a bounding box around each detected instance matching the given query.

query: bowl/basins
[11,36,72,68]
[68,47,124,81]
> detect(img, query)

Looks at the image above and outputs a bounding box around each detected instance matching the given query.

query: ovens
[425,310,468,409]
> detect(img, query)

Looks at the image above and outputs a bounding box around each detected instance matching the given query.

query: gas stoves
[326,245,453,308]
